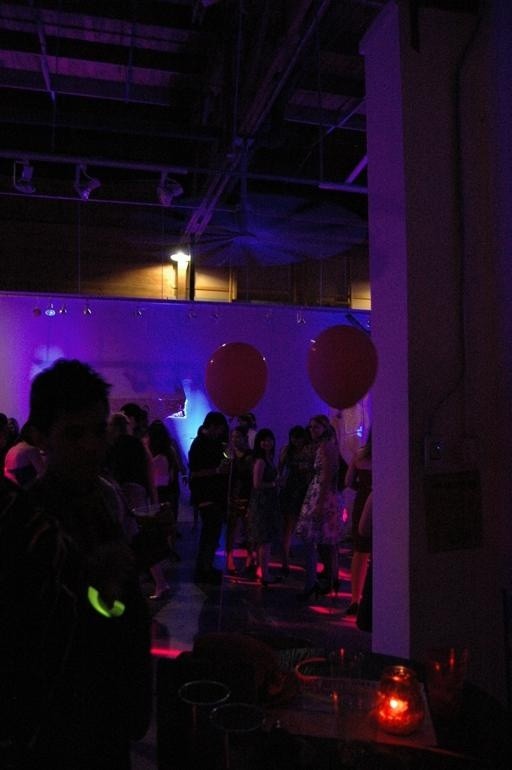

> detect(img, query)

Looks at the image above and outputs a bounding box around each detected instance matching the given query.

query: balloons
[305,323,378,411]
[205,339,270,423]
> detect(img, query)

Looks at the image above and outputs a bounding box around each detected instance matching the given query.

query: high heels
[318,578,342,596]
[296,581,319,602]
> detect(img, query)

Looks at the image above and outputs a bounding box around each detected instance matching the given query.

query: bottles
[373,664,426,735]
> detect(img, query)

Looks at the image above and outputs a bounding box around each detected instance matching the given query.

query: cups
[177,678,267,770]
[129,503,161,528]
